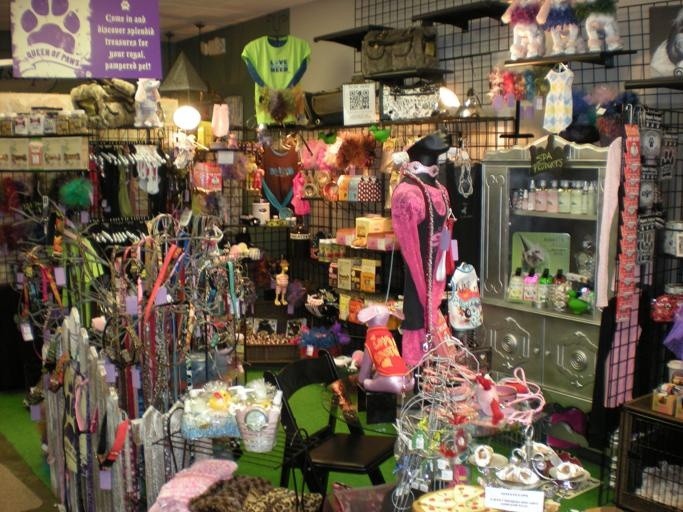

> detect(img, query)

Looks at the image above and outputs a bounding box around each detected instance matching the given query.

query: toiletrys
[507,267,567,302]
[512,177,598,215]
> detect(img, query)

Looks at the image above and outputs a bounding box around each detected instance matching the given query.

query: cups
[666,359,683,386]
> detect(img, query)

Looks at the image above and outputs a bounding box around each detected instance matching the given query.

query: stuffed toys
[501,0,547,60]
[536,0,580,56]
[583,1,625,52]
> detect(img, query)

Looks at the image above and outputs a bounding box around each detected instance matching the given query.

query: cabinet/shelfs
[472,135,621,489]
[0,133,91,173]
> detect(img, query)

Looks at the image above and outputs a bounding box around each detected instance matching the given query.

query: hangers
[88,140,169,167]
[86,215,153,245]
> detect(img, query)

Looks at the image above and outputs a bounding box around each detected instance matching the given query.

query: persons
[391,131,460,364]
[274,258,290,306]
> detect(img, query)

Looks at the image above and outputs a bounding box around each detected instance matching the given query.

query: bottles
[512,179,599,215]
[272,215,297,228]
[28,137,43,164]
[397,294,404,308]
[506,266,567,309]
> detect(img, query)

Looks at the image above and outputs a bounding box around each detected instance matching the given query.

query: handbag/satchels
[360,25,440,77]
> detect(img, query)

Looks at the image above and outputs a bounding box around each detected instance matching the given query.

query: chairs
[262,349,396,511]
